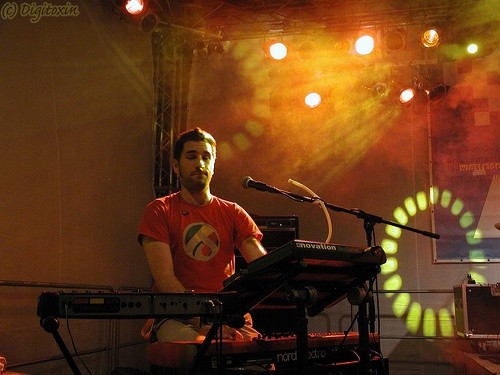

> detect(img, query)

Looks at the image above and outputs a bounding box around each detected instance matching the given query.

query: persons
[138,127,276,375]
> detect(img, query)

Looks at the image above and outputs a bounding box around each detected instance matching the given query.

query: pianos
[37,292,237,375]
[221,239,382,375]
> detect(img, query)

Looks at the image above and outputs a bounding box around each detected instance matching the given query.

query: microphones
[241,176,277,192]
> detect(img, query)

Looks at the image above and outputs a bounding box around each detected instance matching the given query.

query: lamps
[116,0,161,32]
[262,22,480,110]
